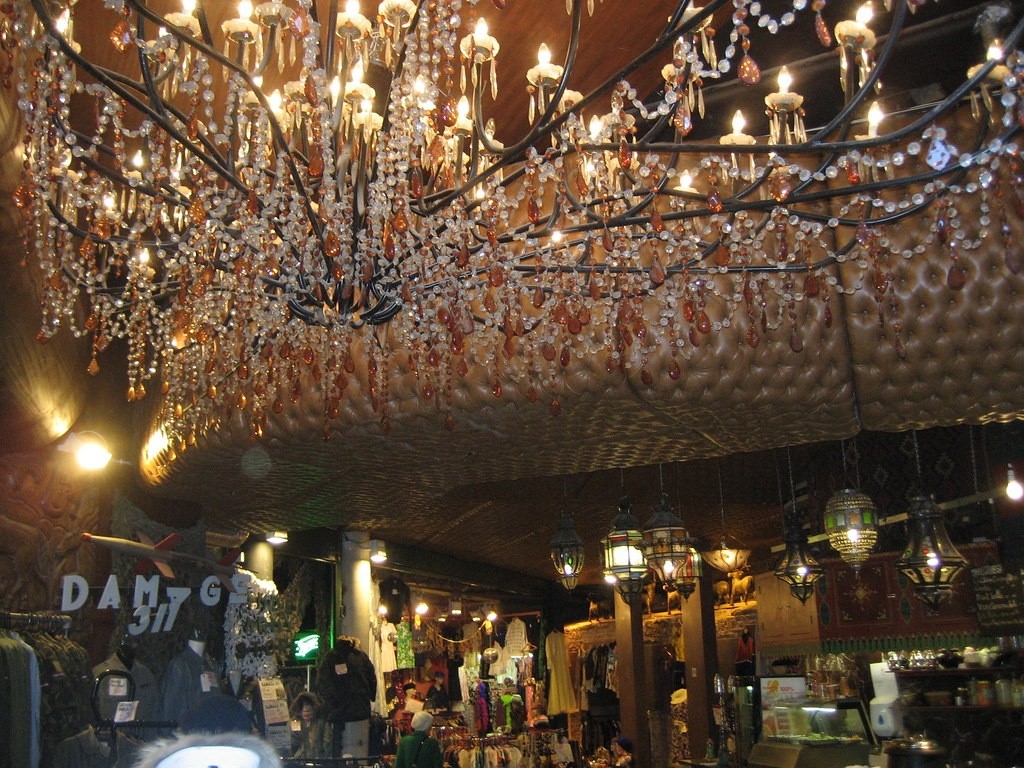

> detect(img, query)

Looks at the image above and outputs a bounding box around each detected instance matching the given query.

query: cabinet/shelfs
[887,667,1024,768]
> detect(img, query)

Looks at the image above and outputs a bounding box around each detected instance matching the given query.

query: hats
[670,688,687,705]
[612,736,633,753]
[410,711,434,731]
[404,683,415,691]
[511,694,522,702]
[435,672,444,677]
[177,695,253,736]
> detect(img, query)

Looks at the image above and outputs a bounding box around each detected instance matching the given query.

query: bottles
[706,739,713,762]
[966,677,1024,707]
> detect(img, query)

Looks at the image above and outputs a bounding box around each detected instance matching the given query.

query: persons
[91,645,159,723]
[611,736,632,767]
[317,636,377,725]
[177,695,253,738]
[402,683,424,713]
[392,711,443,768]
[287,692,332,760]
[427,671,450,711]
[946,718,1024,768]
[160,640,221,721]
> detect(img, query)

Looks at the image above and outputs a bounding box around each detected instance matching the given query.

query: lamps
[600,468,648,610]
[266,531,288,544]
[895,430,970,618]
[451,594,461,614]
[700,459,751,574]
[58,431,113,471]
[0,2,1024,461]
[773,447,824,606]
[411,591,428,615]
[548,476,585,591]
[361,540,387,563]
[823,440,880,580]
[675,532,698,603]
[481,602,497,621]
[634,463,694,593]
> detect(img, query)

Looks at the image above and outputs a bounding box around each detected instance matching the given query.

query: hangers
[521,641,538,652]
[0,610,74,643]
[599,639,605,647]
[553,626,558,632]
[434,726,513,753]
